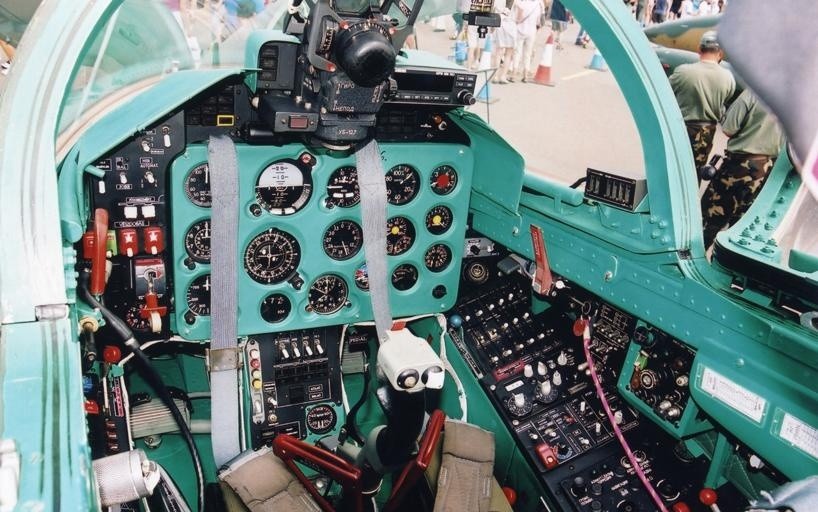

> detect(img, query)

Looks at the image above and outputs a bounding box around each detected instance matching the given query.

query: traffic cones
[528,34,558,88]
[586,46,609,73]
[472,35,502,104]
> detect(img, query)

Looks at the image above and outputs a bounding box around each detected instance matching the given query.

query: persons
[701,88,786,263]
[179,0,260,43]
[625,0,726,26]
[667,29,737,190]
[0,40,17,70]
[453,0,590,83]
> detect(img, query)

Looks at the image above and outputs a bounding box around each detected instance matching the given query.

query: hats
[698,30,720,50]
